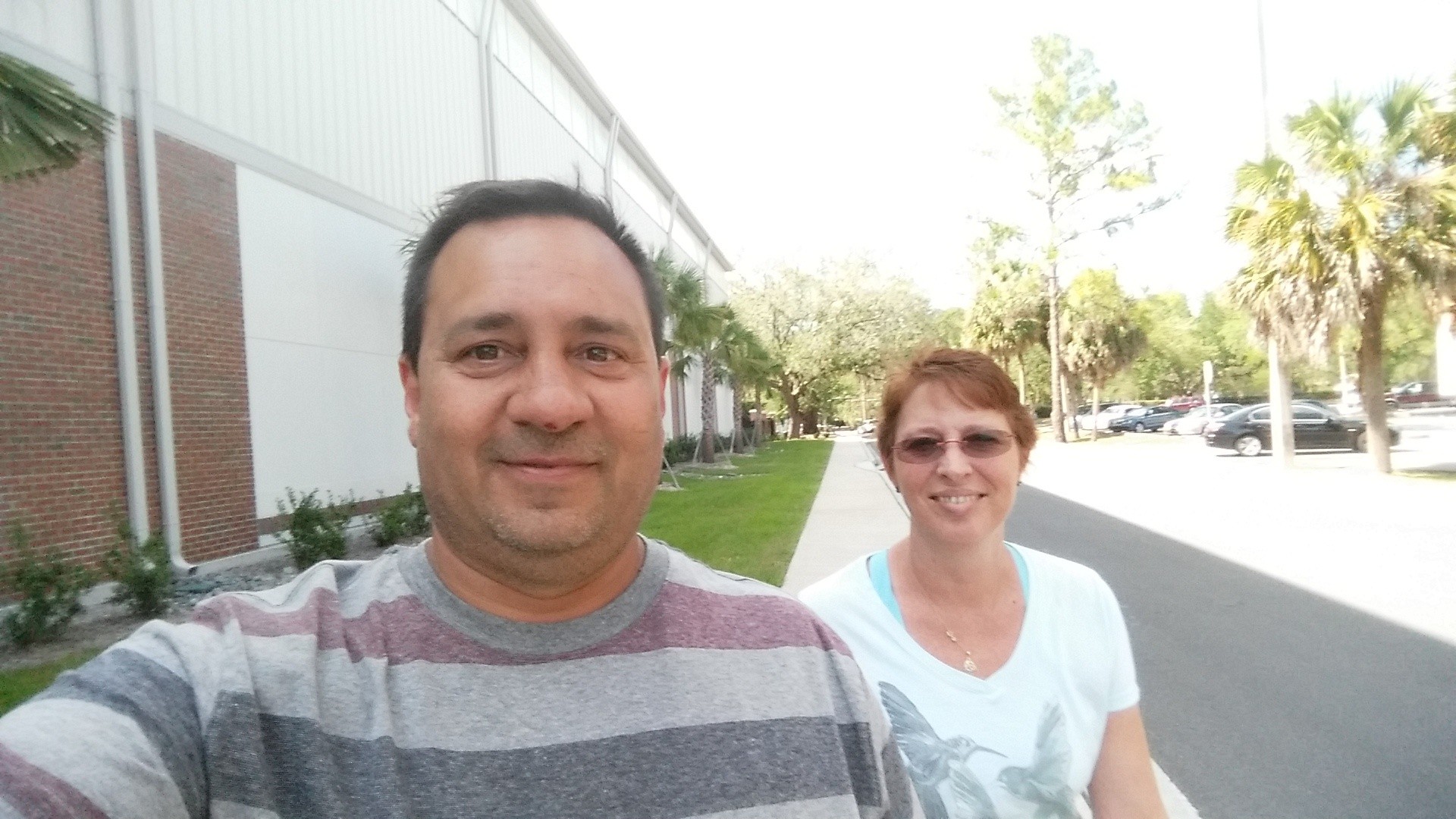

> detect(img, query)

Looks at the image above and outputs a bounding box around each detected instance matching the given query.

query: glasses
[890,430,1017,465]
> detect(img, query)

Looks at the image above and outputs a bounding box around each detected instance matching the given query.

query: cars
[1202,400,1402,457]
[1065,396,1249,440]
[1292,399,1340,414]
[1382,381,1439,411]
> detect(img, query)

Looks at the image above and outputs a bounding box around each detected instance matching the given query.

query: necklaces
[917,574,1003,672]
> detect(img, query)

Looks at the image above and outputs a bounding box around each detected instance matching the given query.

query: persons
[797,348,1170,819]
[0,179,914,819]
